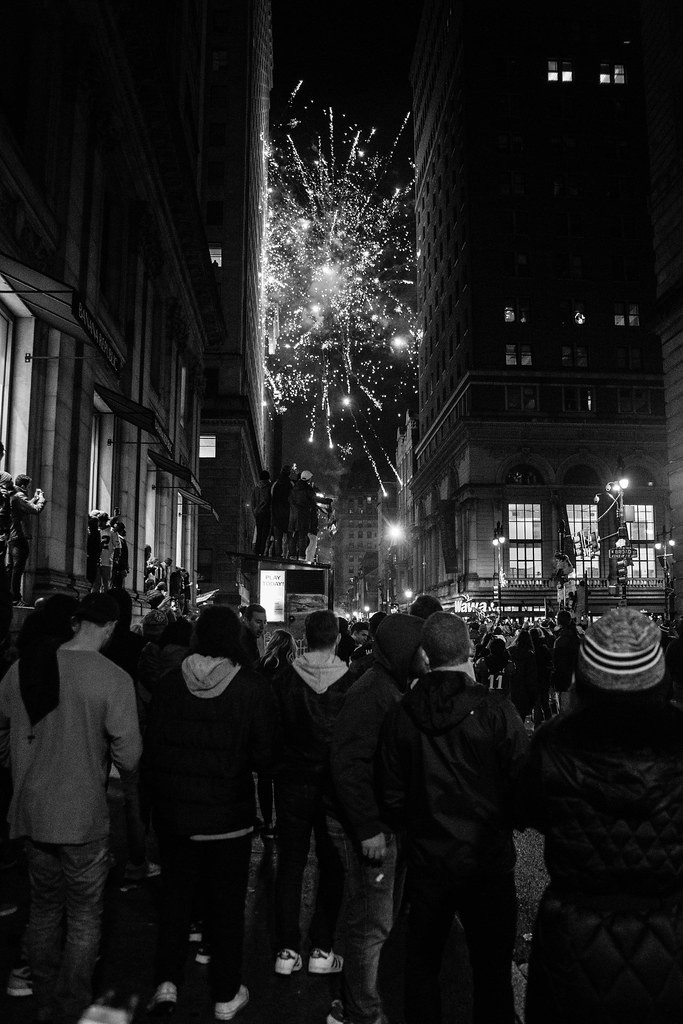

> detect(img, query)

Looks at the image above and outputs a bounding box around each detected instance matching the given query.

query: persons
[0,441,45,609]
[468,610,580,730]
[379,611,530,1024]
[87,509,128,593]
[98,589,298,1021]
[250,465,333,561]
[0,589,143,1024]
[264,596,443,974]
[512,606,683,1024]
[659,616,683,705]
[554,553,573,589]
[145,545,191,615]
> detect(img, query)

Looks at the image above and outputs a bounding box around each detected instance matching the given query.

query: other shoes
[214,984,249,1020]
[189,920,207,942]
[75,984,139,1024]
[0,903,18,917]
[326,999,344,1024]
[147,981,177,1016]
[124,856,161,881]
[12,599,35,609]
[0,856,18,869]
[195,942,211,965]
[6,967,33,997]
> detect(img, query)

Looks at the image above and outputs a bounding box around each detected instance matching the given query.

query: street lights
[492,520,507,625]
[605,457,633,607]
[654,524,676,618]
[405,588,413,616]
[364,606,370,625]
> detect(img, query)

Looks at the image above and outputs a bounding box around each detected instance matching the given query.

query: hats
[579,607,665,692]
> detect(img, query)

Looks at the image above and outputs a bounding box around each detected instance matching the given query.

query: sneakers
[307,947,344,973]
[274,949,304,975]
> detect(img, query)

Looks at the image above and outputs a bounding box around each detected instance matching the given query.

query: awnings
[94,382,174,459]
[178,488,219,522]
[147,449,201,497]
[0,249,128,374]
